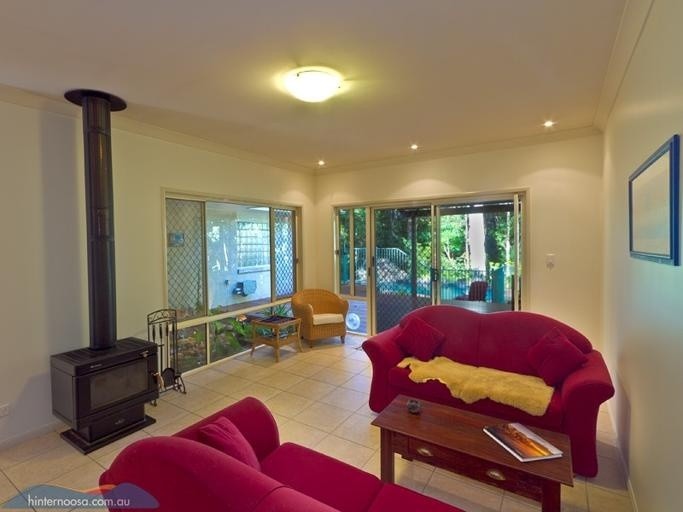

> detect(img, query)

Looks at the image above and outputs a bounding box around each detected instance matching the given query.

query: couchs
[361,305,614,478]
[98,397,466,512]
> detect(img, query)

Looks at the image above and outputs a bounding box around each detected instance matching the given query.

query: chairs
[292,289,349,348]
[456,281,488,300]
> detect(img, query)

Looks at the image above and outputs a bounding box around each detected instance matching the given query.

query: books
[480,420,565,463]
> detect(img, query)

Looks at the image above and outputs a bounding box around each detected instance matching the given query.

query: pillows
[529,328,587,388]
[199,415,261,471]
[395,316,447,362]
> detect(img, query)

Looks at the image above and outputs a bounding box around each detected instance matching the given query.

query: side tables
[250,316,304,362]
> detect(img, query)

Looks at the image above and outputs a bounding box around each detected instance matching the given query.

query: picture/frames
[628,134,680,266]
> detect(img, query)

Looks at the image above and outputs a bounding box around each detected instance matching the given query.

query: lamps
[280,70,342,101]
